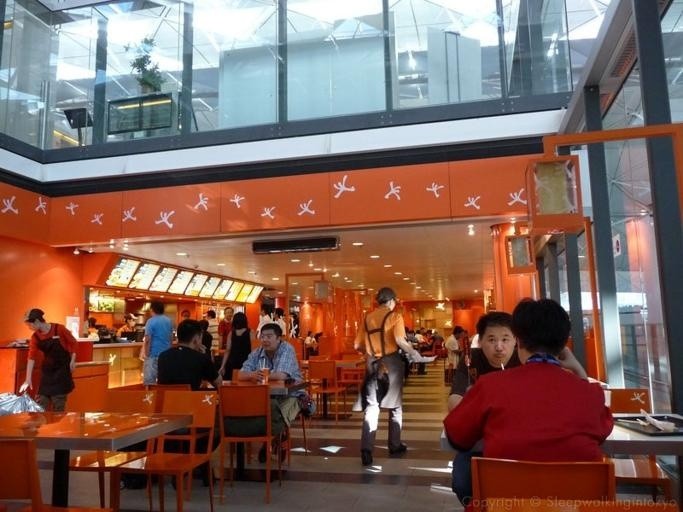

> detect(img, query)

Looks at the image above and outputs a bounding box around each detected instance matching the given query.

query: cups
[255,359,268,384]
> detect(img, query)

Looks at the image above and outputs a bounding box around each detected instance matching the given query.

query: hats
[376,287,397,301]
[23,308,44,322]
[125,313,138,320]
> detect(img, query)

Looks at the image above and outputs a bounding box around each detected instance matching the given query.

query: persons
[19,309,78,411]
[443,298,613,461]
[405,326,479,374]
[447,312,520,507]
[355,288,423,465]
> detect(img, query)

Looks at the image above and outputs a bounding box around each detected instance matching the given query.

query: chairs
[0,331,681,512]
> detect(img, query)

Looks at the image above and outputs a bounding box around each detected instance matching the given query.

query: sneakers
[361,448,373,465]
[389,443,406,456]
